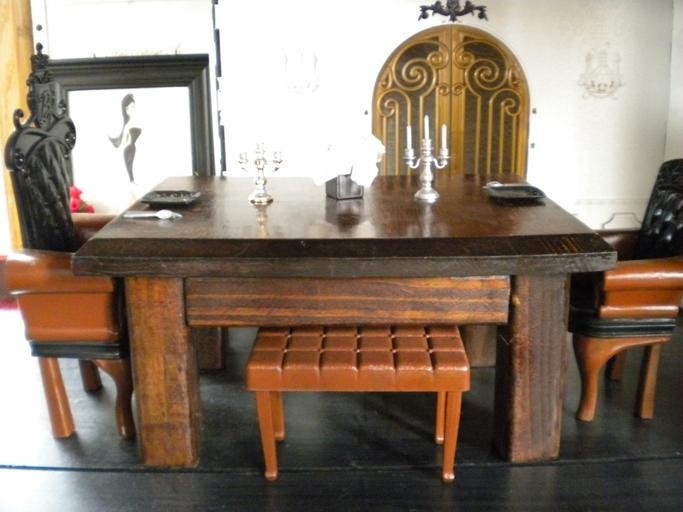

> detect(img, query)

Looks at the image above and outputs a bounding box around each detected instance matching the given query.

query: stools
[246,326,471,487]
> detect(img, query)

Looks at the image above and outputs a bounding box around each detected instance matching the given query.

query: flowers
[63,184,96,214]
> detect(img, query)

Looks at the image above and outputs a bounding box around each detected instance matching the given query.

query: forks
[125,207,183,218]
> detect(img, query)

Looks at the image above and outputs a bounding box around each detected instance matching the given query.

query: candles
[406,125,412,149]
[441,124,447,149]
[424,114,430,141]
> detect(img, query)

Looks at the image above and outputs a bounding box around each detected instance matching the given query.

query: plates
[138,190,200,208]
[486,184,546,208]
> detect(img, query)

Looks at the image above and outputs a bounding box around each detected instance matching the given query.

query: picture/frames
[44,54,213,218]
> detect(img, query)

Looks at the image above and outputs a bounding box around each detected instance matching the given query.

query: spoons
[122,209,171,220]
[479,180,532,189]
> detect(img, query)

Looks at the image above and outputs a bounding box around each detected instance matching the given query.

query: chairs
[568,158,683,423]
[0,42,136,449]
